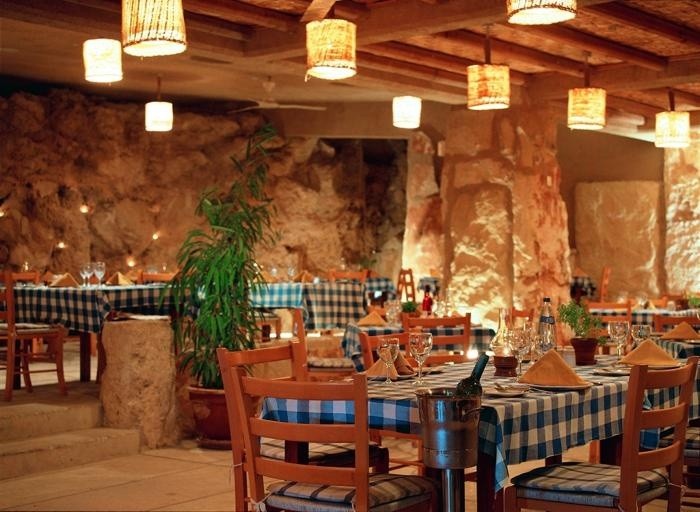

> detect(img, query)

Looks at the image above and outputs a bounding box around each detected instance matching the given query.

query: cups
[631,324,653,346]
[618,289,678,315]
[493,356,518,378]
[314,277,319,283]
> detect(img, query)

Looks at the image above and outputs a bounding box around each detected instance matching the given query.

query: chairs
[356,328,434,478]
[498,354,698,512]
[215,334,391,511]
[232,371,446,510]
[0,261,700,406]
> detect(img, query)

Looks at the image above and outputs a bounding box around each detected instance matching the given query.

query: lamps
[465,21,513,113]
[654,87,692,150]
[565,45,606,133]
[305,0,359,83]
[390,93,422,130]
[77,0,188,133]
[504,0,578,28]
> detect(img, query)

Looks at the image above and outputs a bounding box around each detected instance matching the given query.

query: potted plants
[159,126,277,451]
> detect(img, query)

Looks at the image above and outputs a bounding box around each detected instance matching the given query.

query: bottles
[490,307,516,357]
[554,344,577,368]
[287,266,296,283]
[454,354,490,396]
[271,266,277,277]
[423,284,432,316]
[538,298,557,353]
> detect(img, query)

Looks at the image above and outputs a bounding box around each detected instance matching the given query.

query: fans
[221,72,325,116]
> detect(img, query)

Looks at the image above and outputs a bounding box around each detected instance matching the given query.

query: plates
[592,368,631,376]
[364,373,418,381]
[658,336,700,345]
[415,367,444,374]
[486,387,524,398]
[509,381,595,390]
[631,363,683,371]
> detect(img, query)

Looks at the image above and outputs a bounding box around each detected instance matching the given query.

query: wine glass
[607,320,630,361]
[407,332,435,387]
[383,299,403,326]
[78,259,106,290]
[377,338,401,385]
[509,321,537,376]
